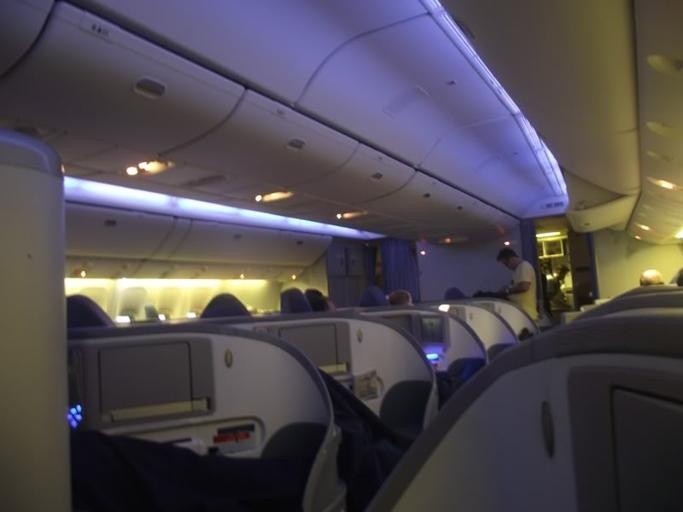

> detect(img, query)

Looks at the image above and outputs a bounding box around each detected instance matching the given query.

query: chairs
[65,287,537,511]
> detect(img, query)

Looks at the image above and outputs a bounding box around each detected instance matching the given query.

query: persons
[640,269,664,286]
[307,294,329,312]
[495,247,538,323]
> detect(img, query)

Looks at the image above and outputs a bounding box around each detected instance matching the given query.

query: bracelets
[509,289,513,294]
